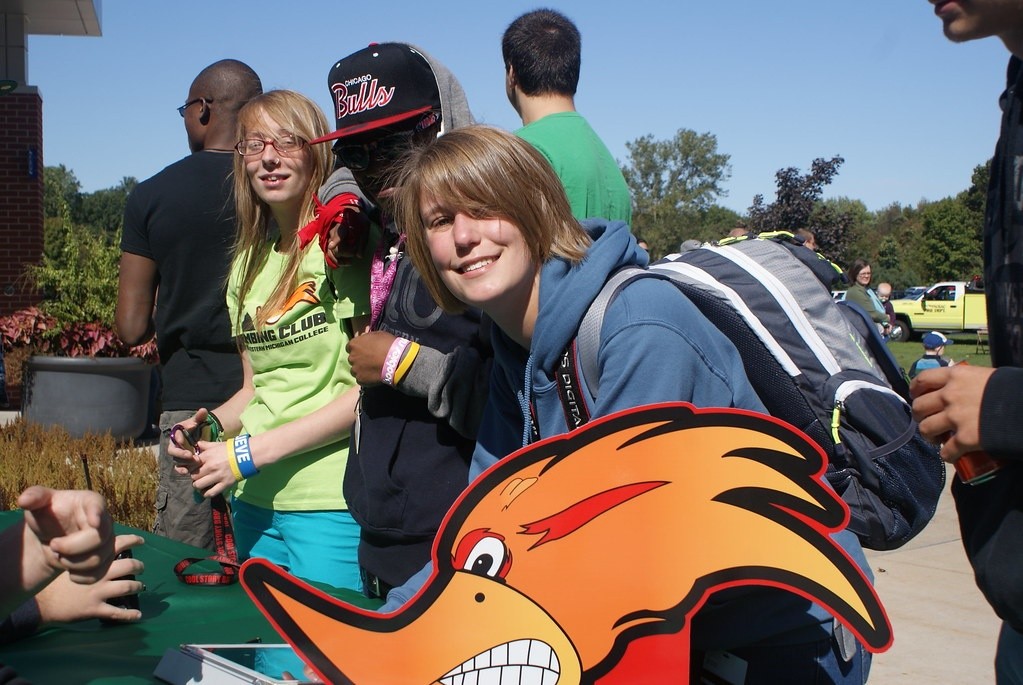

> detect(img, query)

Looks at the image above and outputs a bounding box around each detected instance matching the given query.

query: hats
[680,240,702,252]
[735,219,746,228]
[308,41,442,147]
[923,330,954,349]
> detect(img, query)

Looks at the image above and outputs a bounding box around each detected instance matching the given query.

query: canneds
[939,360,1002,486]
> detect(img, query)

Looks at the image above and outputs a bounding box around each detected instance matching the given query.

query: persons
[502,7,632,232]
[907,330,953,381]
[167,91,364,597]
[875,282,896,333]
[680,238,702,253]
[393,124,872,685]
[115,58,263,553]
[726,228,750,238]
[909,0,1023,685]
[796,229,815,252]
[637,239,649,254]
[309,41,496,606]
[845,258,893,344]
[1,483,146,685]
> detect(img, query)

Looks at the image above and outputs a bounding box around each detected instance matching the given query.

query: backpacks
[554,230,947,552]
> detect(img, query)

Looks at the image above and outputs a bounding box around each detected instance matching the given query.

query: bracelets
[380,337,420,385]
[227,434,260,482]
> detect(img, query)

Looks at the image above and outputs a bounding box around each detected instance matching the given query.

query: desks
[0,506,388,685]
[976,330,988,354]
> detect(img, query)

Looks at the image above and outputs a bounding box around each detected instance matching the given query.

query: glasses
[332,112,441,173]
[234,135,308,155]
[177,97,213,118]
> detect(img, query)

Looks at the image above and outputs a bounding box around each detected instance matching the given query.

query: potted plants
[20,192,151,445]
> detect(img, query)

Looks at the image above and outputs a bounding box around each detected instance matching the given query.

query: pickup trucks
[831,282,988,342]
